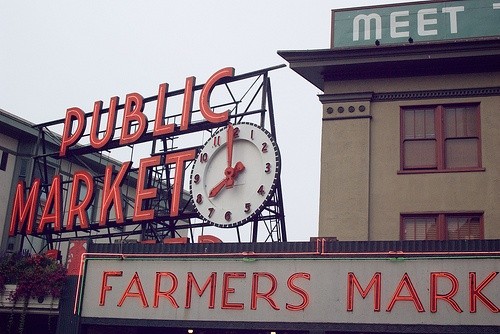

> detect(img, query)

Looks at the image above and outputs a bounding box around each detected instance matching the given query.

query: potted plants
[0,248,68,309]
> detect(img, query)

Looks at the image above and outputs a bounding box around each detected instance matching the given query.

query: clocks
[189,122,282,228]
